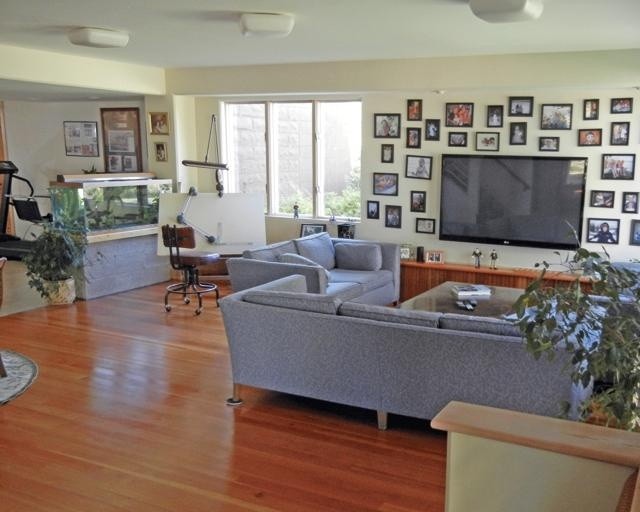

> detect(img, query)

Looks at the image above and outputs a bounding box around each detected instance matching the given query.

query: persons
[489,249,498,270]
[152,113,168,134]
[368,99,640,245]
[340,226,348,238]
[471,248,484,269]
[293,202,300,220]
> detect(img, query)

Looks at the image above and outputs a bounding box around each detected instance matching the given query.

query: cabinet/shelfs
[399,260,591,306]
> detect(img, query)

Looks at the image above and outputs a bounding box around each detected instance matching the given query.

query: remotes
[470,301,477,306]
[464,301,474,311]
[456,301,465,309]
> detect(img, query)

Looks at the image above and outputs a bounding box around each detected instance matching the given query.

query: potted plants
[23,204,112,305]
[507,223,640,434]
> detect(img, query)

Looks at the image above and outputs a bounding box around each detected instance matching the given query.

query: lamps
[238,11,295,38]
[69,28,129,48]
[183,113,228,197]
[470,0,543,21]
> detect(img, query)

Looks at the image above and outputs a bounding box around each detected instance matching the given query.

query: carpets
[0,345,39,406]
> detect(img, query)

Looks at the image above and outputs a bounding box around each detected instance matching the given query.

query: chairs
[11,191,53,241]
[0,256,9,378]
[156,225,221,317]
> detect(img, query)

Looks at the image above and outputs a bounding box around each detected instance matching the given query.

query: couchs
[217,273,585,434]
[226,232,401,312]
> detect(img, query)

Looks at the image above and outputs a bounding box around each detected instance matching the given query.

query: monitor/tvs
[439,154,588,249]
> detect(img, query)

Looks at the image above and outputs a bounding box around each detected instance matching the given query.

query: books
[450,285,491,300]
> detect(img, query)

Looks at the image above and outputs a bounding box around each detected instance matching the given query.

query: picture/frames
[404,153,433,182]
[487,104,505,129]
[414,216,436,235]
[609,97,634,115]
[600,152,636,182]
[404,126,422,149]
[578,128,602,147]
[444,101,475,129]
[474,131,501,153]
[628,219,640,246]
[424,117,442,141]
[299,223,326,238]
[621,190,639,214]
[372,111,402,139]
[99,105,143,174]
[148,111,171,136]
[538,135,560,153]
[153,142,169,161]
[365,200,380,220]
[588,188,616,210]
[610,121,631,147]
[409,190,427,214]
[582,98,600,121]
[508,95,534,118]
[380,143,395,164]
[447,130,468,148]
[508,121,529,146]
[586,216,621,246]
[540,103,574,131]
[62,119,101,157]
[406,97,423,122]
[372,172,399,198]
[384,204,403,230]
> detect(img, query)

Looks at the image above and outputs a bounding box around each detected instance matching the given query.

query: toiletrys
[424,251,440,263]
